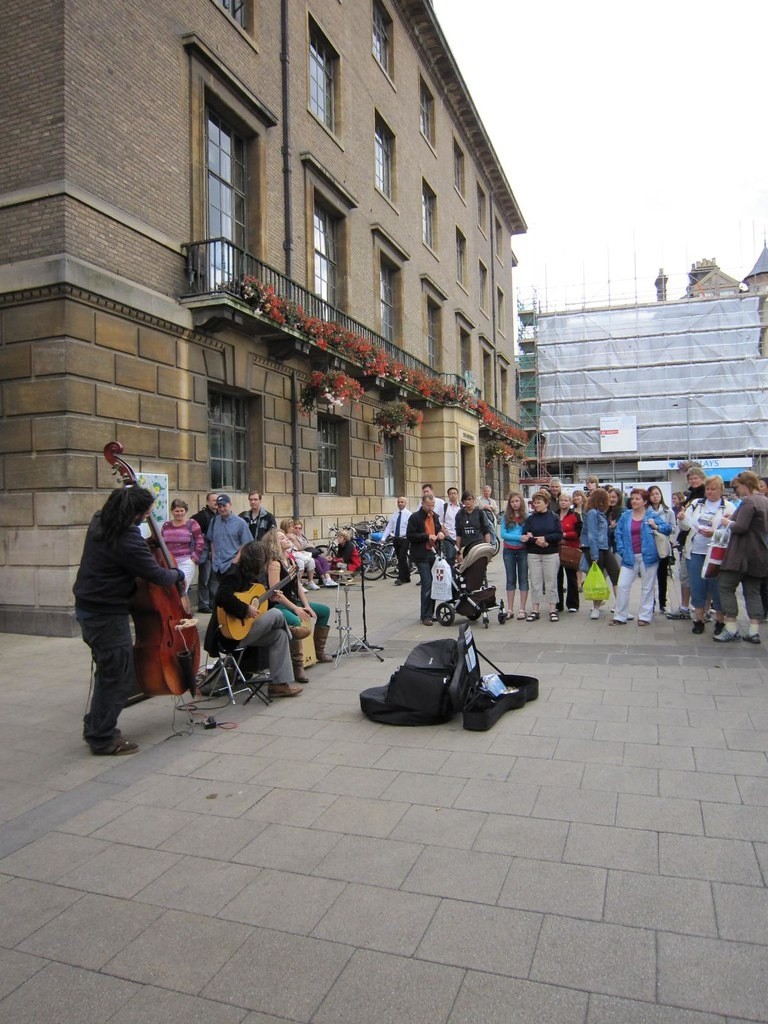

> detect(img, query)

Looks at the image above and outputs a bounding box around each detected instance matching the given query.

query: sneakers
[421,594,762,645]
[323,579,338,587]
[308,583,320,590]
[301,584,308,593]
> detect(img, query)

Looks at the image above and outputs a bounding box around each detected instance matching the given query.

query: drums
[289,613,316,668]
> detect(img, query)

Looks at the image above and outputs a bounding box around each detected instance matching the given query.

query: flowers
[225,278,530,465]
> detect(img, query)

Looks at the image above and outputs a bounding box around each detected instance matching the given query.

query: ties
[395,512,401,539]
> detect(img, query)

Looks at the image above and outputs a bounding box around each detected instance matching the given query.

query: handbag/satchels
[560,546,582,569]
[430,557,453,601]
[652,530,672,558]
[701,521,734,580]
[583,561,611,601]
[305,547,320,558]
[187,519,208,565]
[386,638,458,714]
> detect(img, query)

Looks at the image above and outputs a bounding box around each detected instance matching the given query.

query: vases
[315,391,332,404]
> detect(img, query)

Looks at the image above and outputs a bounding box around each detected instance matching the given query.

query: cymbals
[336,580,360,583]
[326,570,353,575]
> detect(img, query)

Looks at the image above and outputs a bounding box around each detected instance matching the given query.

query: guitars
[216,565,300,641]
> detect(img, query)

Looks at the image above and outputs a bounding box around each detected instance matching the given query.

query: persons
[416,483,499,586]
[501,493,531,620]
[539,467,768,622]
[237,490,277,541]
[266,530,333,683]
[712,470,768,643]
[524,490,562,623]
[160,498,204,616]
[188,492,220,614]
[676,475,736,634]
[204,541,311,698]
[331,528,362,581]
[280,519,338,594]
[206,494,254,581]
[380,496,412,586]
[608,489,672,626]
[73,487,187,755]
[406,495,445,626]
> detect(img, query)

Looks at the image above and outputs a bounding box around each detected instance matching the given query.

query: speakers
[121,642,153,707]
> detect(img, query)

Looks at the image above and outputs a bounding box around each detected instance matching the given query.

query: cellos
[102,437,201,723]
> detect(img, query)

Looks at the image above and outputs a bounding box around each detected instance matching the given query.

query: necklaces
[465,508,474,523]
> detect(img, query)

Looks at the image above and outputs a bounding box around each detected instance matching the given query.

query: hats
[216,494,230,505]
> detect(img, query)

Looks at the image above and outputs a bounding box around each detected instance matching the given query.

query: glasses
[464,497,472,500]
[295,527,301,529]
[731,485,740,492]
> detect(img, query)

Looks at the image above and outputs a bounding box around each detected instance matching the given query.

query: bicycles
[314,514,418,580]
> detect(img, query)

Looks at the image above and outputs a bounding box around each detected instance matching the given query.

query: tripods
[334,549,384,669]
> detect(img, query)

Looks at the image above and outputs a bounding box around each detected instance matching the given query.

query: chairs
[194,610,254,704]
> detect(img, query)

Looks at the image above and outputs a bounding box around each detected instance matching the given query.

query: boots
[289,640,309,682]
[314,623,334,662]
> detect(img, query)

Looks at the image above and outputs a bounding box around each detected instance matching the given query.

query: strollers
[428,542,507,628]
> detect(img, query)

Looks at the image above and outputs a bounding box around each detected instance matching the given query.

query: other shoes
[289,625,311,639]
[395,579,402,585]
[90,738,140,755]
[267,684,303,698]
[82,727,121,743]
[198,608,212,613]
